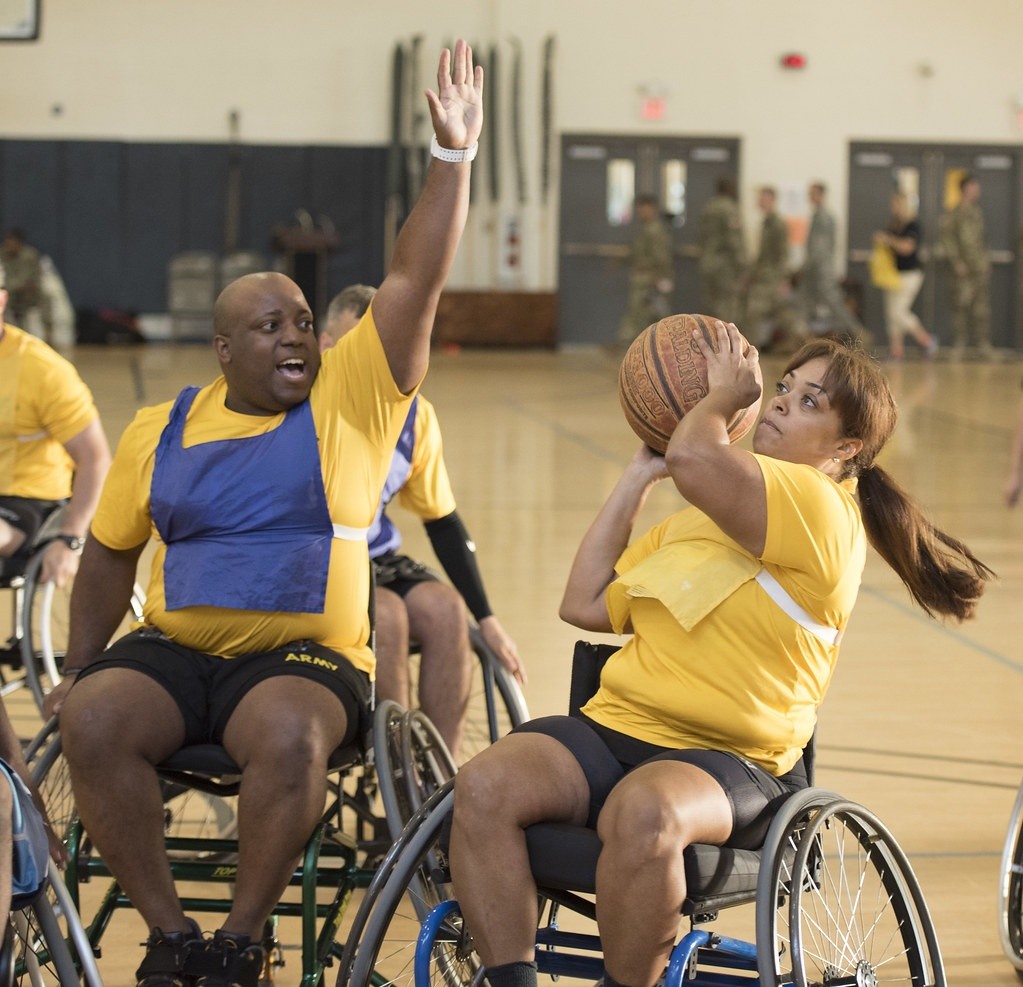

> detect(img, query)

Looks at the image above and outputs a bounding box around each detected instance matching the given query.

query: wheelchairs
[0,480,948,985]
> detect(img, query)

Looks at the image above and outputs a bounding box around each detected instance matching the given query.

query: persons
[0,266,112,590]
[937,176,1006,364]
[40,38,488,987]
[4,227,78,351]
[801,184,874,354]
[1,696,65,987]
[875,189,941,363]
[442,332,1003,987]
[316,281,530,793]
[696,178,750,329]
[741,186,788,351]
[601,193,674,357]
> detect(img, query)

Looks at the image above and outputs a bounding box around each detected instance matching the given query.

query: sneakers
[131,916,200,987]
[193,932,275,984]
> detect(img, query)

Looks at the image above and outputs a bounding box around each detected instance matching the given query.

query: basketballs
[616,312,764,455]
